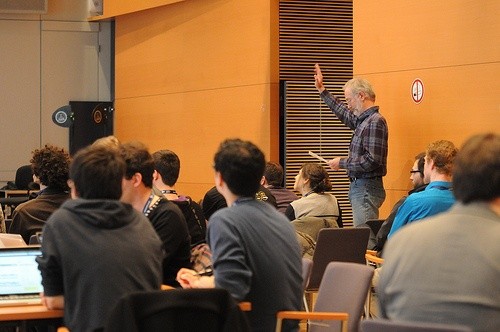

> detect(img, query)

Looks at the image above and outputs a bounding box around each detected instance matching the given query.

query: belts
[349,176,382,182]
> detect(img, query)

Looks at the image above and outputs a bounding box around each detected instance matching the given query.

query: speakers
[68,101,113,157]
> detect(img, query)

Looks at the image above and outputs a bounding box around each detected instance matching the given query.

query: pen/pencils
[175,268,213,282]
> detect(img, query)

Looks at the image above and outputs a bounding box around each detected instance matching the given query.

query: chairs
[0,189,500,332]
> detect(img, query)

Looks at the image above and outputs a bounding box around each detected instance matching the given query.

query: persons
[9,134,343,332]
[376,133,500,332]
[314,63,388,252]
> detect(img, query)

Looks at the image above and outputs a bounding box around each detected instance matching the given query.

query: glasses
[410,169,421,174]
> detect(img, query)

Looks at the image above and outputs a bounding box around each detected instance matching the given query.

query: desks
[0,284,252,320]
